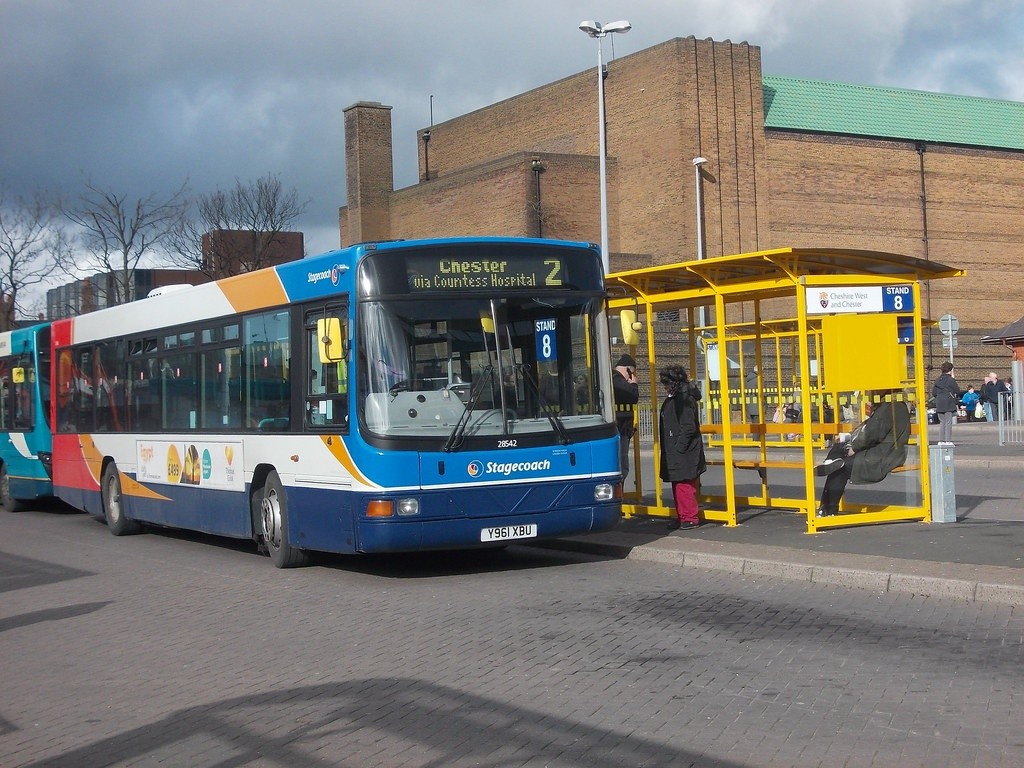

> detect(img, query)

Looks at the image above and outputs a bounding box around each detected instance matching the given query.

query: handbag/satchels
[952,396,959,405]
[975,402,983,418]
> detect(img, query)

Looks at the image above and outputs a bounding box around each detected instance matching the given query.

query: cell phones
[627,369,632,379]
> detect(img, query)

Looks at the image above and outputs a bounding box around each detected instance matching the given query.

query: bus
[0,322,63,513]
[50,234,625,570]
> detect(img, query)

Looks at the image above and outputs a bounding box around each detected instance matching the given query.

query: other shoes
[805,509,836,525]
[667,519,682,530]
[681,519,700,529]
[813,456,845,477]
[938,441,956,447]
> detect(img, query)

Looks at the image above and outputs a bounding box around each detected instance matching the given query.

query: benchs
[700,423,920,473]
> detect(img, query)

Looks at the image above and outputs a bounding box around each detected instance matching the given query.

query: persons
[979,373,1014,422]
[746,365,768,441]
[574,374,590,414]
[612,354,639,516]
[806,388,911,524]
[59,389,108,432]
[773,384,871,448]
[539,360,560,413]
[962,385,980,422]
[658,364,707,530]
[494,374,519,408]
[932,362,960,447]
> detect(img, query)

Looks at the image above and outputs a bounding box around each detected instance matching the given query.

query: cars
[922,388,986,423]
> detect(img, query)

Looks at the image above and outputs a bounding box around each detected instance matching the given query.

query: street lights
[579,19,633,275]
[692,156,707,340]
[422,129,429,183]
[532,158,543,239]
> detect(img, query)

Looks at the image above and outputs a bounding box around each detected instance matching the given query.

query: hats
[616,353,637,368]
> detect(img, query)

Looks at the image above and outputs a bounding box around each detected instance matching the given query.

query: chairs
[71,376,289,434]
